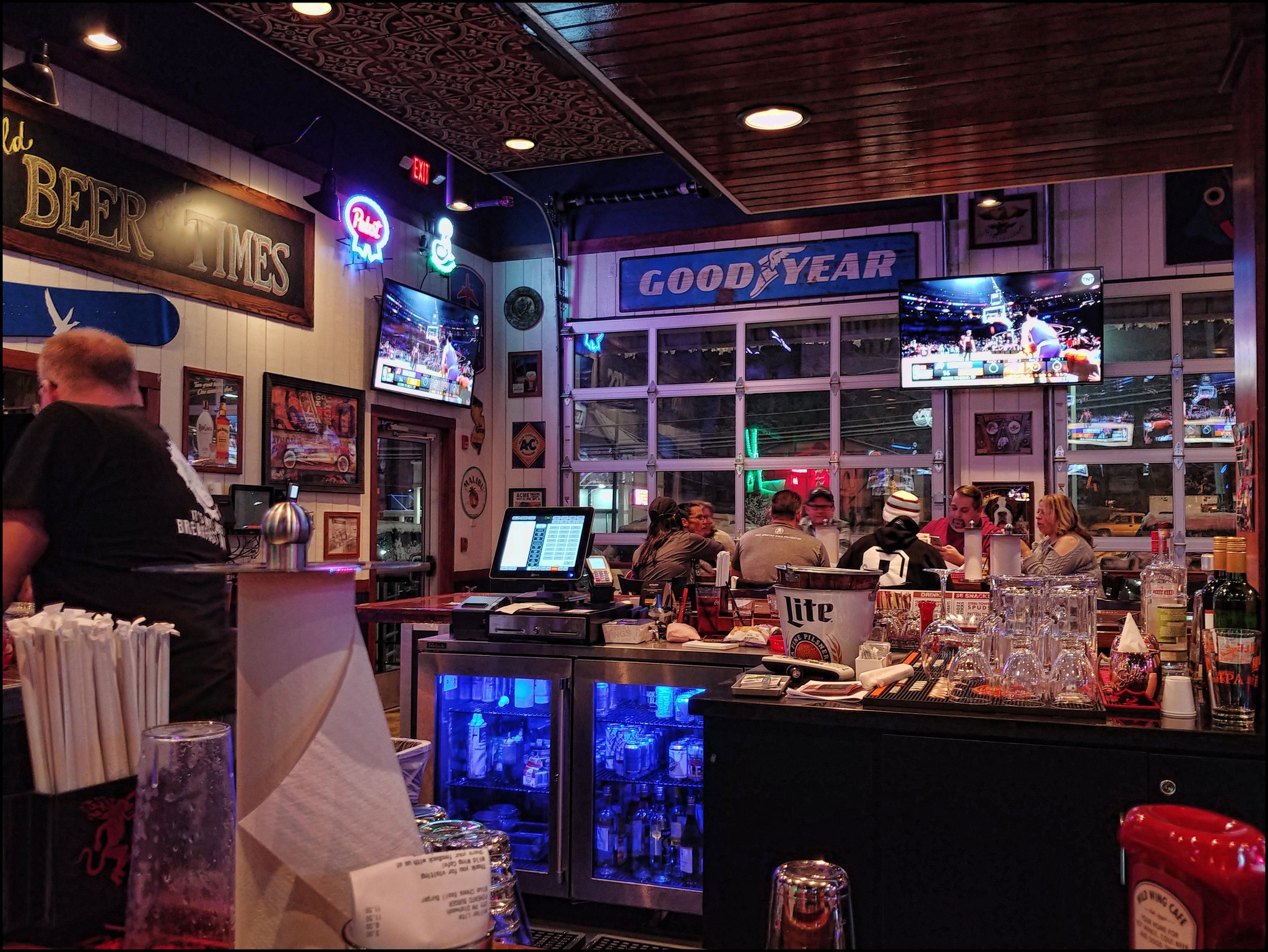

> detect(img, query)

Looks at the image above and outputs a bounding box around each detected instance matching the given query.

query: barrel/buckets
[773,580,882,671]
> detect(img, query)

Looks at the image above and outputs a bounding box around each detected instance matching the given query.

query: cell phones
[285,482,300,503]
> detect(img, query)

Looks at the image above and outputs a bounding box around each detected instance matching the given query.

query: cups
[872,611,919,649]
[341,914,497,949]
[411,803,534,949]
[768,593,780,619]
[855,652,893,683]
[696,588,721,634]
[732,597,754,628]
[1201,630,1262,726]
[1160,674,1198,719]
[122,720,236,949]
[630,597,641,608]
[992,576,1161,703]
[764,859,854,951]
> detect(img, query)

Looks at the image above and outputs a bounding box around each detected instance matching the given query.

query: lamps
[400,155,446,187]
[252,113,343,223]
[446,152,473,212]
[974,189,1006,208]
[3,35,59,106]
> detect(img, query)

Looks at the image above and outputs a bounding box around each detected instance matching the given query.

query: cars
[1088,512,1146,537]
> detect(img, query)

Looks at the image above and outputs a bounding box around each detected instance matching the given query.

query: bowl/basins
[266,542,306,571]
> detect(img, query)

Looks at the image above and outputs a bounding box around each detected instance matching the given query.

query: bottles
[216,395,229,465]
[1140,529,1260,705]
[467,714,521,833]
[594,783,703,887]
[1118,805,1268,951]
[196,400,214,458]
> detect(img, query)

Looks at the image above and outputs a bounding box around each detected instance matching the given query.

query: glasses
[32,383,57,417]
[690,515,706,521]
[808,504,833,511]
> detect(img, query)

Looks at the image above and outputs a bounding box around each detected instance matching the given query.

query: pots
[774,562,883,590]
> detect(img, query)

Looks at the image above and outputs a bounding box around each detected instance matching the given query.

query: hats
[809,486,834,503]
[649,497,688,518]
[882,491,920,524]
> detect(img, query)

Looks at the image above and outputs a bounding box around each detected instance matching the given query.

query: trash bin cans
[390,737,432,805]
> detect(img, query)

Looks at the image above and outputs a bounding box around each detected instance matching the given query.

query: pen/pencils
[672,590,678,613]
[657,593,662,608]
[243,526,260,528]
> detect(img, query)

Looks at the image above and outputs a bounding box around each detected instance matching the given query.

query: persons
[798,486,850,568]
[1002,493,1107,599]
[729,489,831,586]
[1143,379,1255,476]
[676,503,718,576]
[631,496,725,584]
[689,499,736,562]
[2,326,239,729]
[378,333,474,407]
[1067,407,1135,447]
[900,301,1101,383]
[1236,478,1253,532]
[836,490,955,592]
[915,485,1005,569]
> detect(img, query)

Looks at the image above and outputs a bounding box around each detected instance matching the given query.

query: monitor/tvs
[369,278,483,409]
[490,507,595,603]
[229,484,274,536]
[898,266,1104,391]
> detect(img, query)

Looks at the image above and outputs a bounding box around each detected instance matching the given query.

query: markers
[678,586,690,625]
[665,611,670,625]
[663,594,670,608]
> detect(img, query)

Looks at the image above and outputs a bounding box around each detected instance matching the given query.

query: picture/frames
[966,192,1038,250]
[973,411,1034,456]
[971,481,1035,550]
[507,351,543,398]
[508,487,546,507]
[183,365,244,475]
[323,510,361,559]
[261,371,372,495]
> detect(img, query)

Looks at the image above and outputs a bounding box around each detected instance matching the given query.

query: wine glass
[921,569,967,684]
[947,629,1101,708]
